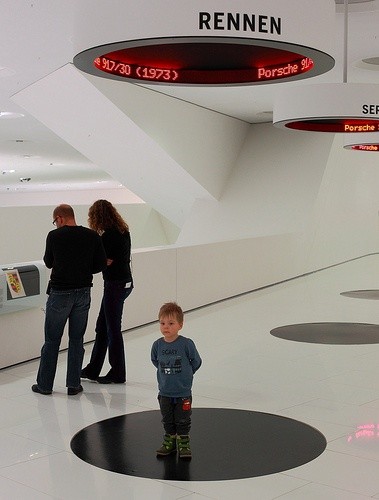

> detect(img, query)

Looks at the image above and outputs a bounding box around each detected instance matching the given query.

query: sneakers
[176,434,192,458]
[154,432,177,456]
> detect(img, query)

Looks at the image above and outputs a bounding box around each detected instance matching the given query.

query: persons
[32,200,134,395]
[151,302,202,457]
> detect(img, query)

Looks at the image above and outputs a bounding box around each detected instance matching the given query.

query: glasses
[52,218,59,227]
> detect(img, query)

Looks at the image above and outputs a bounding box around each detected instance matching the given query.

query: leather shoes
[31,384,53,395]
[68,385,83,395]
[96,375,126,384]
[80,372,99,380]
